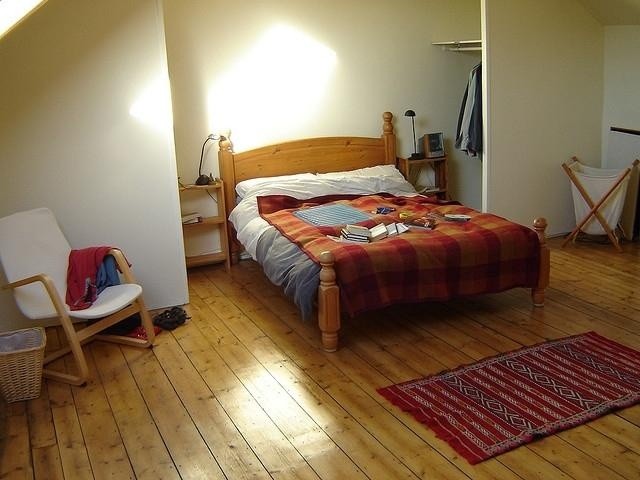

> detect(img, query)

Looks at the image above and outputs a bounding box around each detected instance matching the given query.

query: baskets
[0,326,48,405]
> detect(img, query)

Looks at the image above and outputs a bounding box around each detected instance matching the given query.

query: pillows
[234,164,417,207]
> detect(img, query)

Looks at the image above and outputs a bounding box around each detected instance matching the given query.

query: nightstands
[396,152,448,201]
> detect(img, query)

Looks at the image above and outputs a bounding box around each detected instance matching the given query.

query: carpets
[376,331,639,466]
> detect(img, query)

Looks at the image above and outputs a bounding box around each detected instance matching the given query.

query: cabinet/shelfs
[179,177,230,272]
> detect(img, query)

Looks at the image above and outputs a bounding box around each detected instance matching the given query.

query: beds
[217,112,549,352]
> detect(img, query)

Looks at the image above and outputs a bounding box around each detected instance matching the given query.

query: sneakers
[152,307,187,332]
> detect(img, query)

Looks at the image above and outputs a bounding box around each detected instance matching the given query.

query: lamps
[405,110,424,160]
[195,133,219,185]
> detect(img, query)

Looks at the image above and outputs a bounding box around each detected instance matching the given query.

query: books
[327,222,434,244]
[442,213,472,222]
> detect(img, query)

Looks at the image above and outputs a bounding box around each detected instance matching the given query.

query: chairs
[0,206,156,386]
